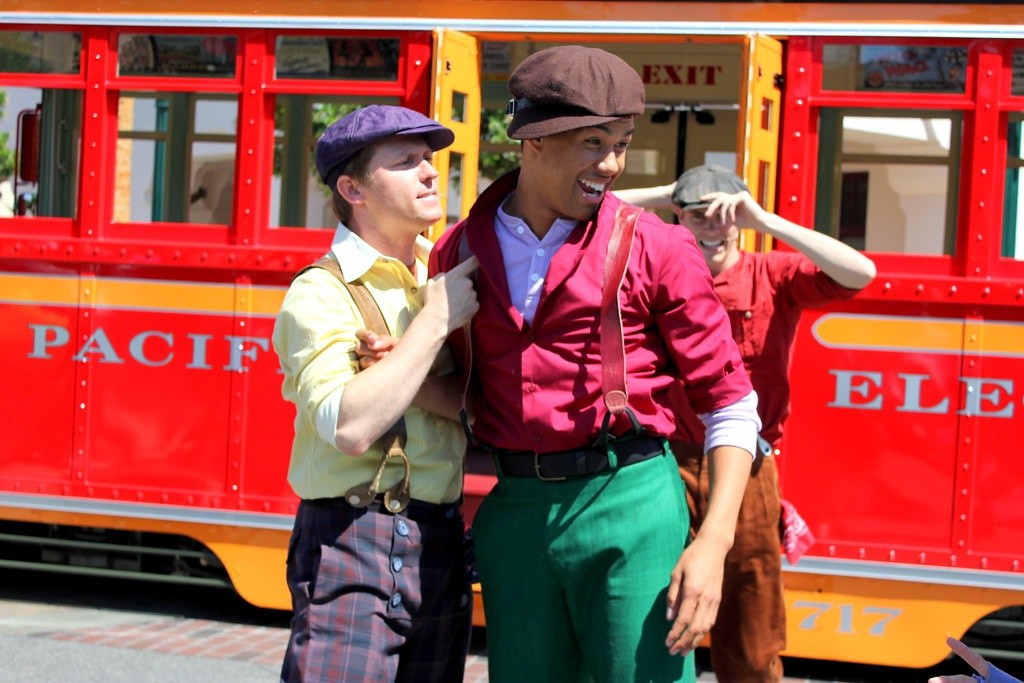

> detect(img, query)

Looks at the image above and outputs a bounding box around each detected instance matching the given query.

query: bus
[0,3,1022,675]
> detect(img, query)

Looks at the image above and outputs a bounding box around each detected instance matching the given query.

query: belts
[491,430,664,482]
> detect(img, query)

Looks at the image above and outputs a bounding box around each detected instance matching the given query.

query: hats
[507,44,645,140]
[316,104,456,190]
[671,163,751,212]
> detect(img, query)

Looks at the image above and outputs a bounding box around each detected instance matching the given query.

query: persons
[266,100,480,683]
[606,165,875,683]
[351,45,766,683]
